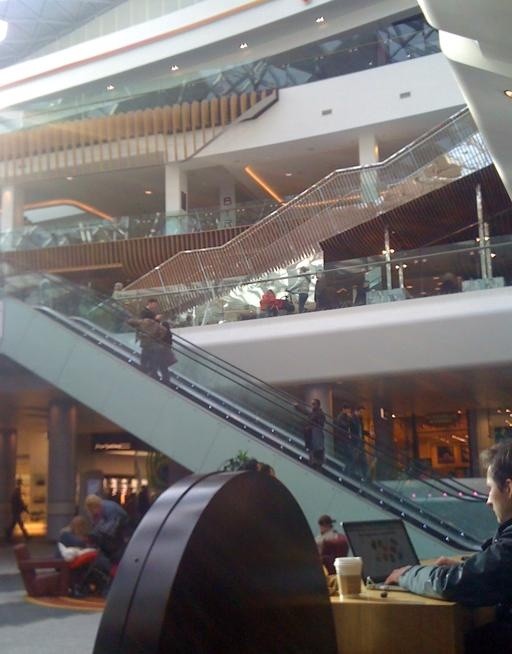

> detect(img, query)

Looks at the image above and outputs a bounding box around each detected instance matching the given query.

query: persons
[311,514,350,574]
[288,265,310,313]
[56,484,156,595]
[294,398,375,484]
[8,480,31,542]
[387,436,511,651]
[314,266,372,311]
[149,323,172,382]
[139,299,161,366]
[111,281,127,334]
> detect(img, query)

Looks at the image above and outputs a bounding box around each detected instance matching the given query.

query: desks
[323,552,497,654]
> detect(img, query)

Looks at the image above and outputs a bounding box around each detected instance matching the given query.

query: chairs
[14,540,74,598]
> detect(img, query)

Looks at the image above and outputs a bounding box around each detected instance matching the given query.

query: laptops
[340,519,422,592]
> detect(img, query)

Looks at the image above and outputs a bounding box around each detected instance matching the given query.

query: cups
[334,556,363,598]
